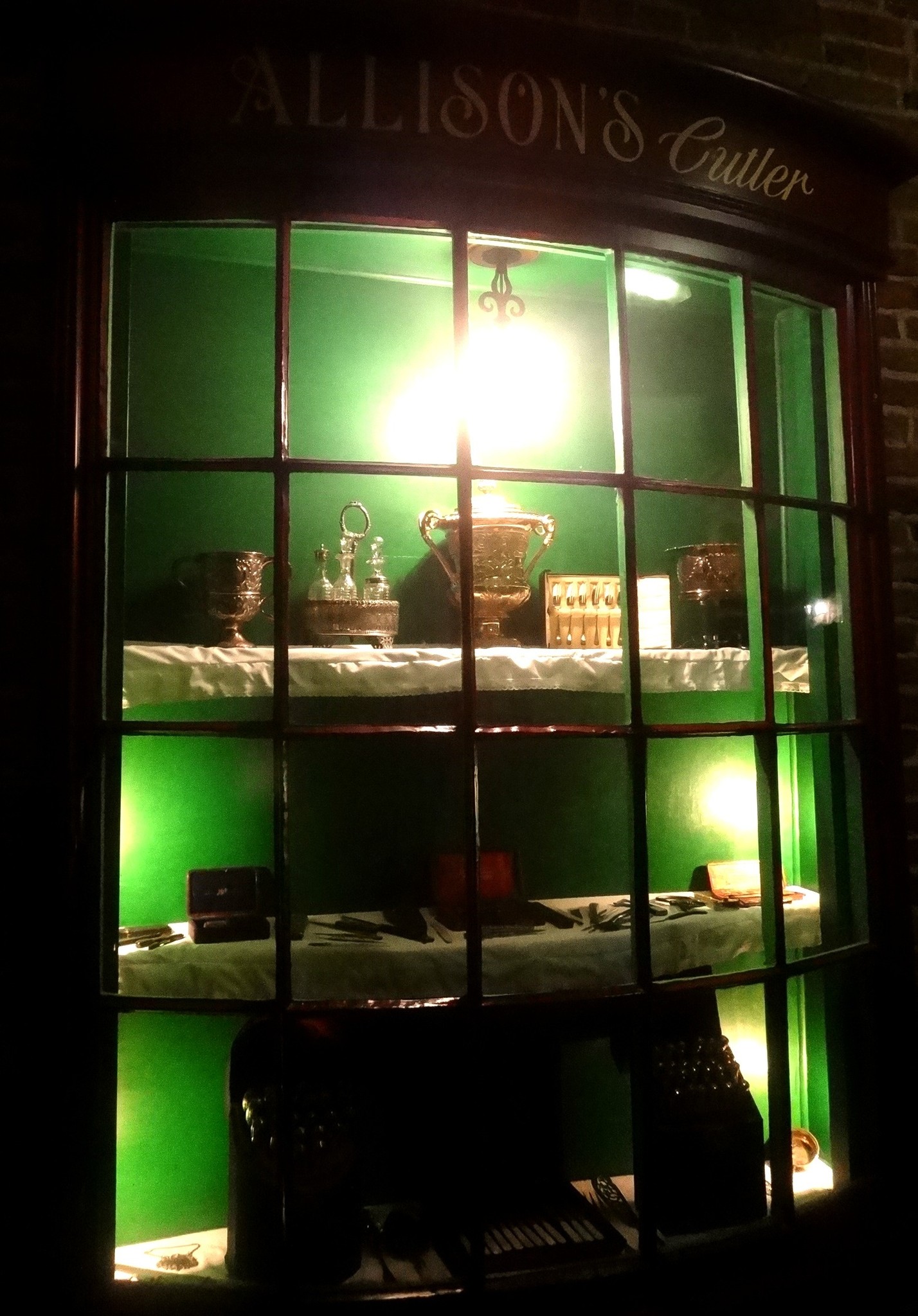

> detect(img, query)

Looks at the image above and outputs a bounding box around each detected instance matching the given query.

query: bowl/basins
[792,1127,820,1172]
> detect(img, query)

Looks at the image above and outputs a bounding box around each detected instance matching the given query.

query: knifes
[421,908,452,944]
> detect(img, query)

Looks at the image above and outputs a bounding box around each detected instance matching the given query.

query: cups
[172,550,292,651]
[418,479,554,647]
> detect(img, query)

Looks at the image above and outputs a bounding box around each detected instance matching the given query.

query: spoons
[552,583,620,647]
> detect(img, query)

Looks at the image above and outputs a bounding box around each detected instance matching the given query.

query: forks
[583,1191,611,1222]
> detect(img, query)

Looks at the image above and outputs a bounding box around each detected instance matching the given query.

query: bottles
[333,533,390,601]
[308,543,334,600]
